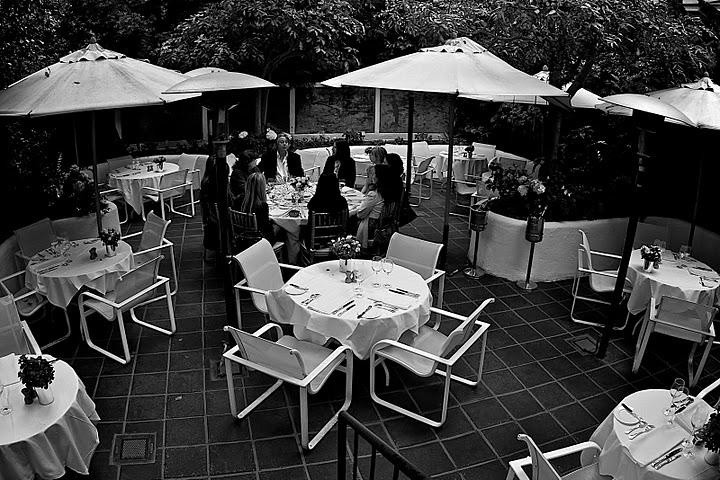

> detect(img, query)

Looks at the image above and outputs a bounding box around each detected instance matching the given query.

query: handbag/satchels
[399,192,417,227]
[375,224,398,245]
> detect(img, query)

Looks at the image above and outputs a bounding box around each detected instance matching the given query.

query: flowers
[640,240,662,269]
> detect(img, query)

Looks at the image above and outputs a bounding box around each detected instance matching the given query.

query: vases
[642,259,654,273]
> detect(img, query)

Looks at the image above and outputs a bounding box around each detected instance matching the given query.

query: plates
[614,406,640,424]
[285,286,306,295]
[358,309,381,319]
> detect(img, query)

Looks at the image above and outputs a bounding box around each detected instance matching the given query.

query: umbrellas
[593,70,720,256]
[457,64,606,111]
[316,37,570,243]
[0,29,203,237]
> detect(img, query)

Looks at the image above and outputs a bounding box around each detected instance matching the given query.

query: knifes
[397,288,420,297]
[655,455,680,470]
[622,403,641,421]
[674,398,694,414]
[332,299,354,315]
[651,448,683,467]
[367,297,407,310]
[338,303,356,317]
[290,284,310,289]
[389,289,419,298]
[37,264,59,274]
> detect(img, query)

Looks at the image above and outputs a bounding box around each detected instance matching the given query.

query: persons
[323,139,356,187]
[361,147,387,193]
[230,149,261,196]
[383,154,417,227]
[261,135,304,179]
[240,172,288,267]
[301,172,349,251]
[201,155,237,250]
[350,165,398,249]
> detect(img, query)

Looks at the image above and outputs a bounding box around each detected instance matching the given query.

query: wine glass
[653,239,666,253]
[664,386,689,427]
[681,406,709,448]
[664,378,685,415]
[371,256,382,287]
[679,246,692,260]
[382,260,393,288]
[682,420,710,459]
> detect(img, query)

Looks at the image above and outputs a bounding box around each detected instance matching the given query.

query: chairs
[696,378,720,408]
[632,296,719,388]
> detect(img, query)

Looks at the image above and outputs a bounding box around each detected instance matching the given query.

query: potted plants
[691,406,720,466]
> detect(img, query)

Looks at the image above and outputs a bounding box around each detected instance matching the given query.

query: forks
[628,424,655,440]
